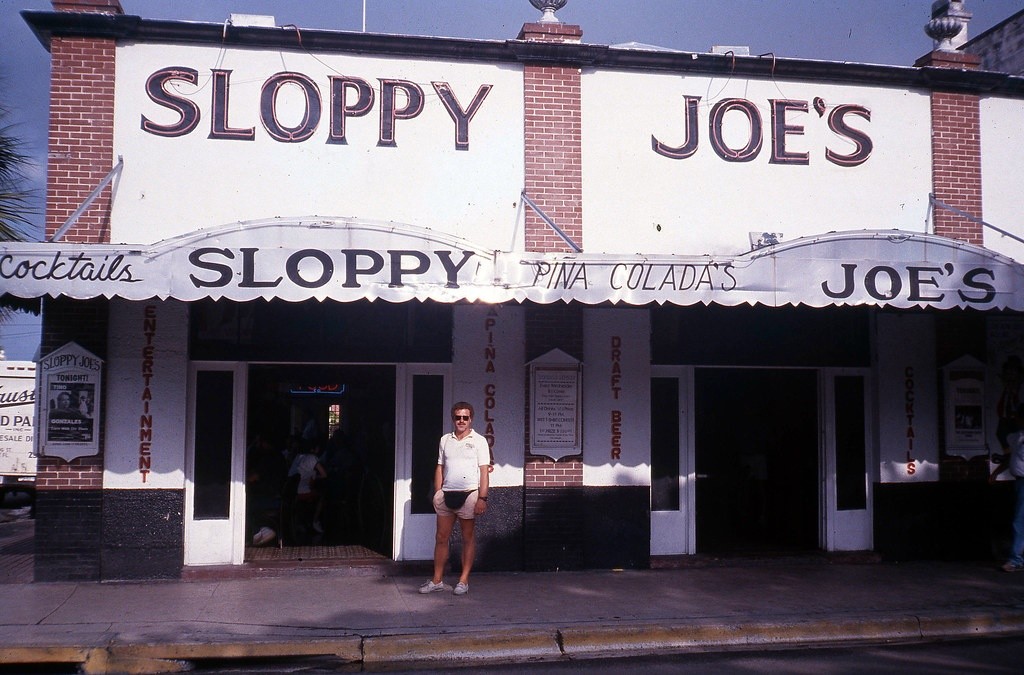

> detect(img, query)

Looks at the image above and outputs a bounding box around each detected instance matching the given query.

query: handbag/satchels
[442,489,477,510]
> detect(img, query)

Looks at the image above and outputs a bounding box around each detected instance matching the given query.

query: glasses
[454,414,471,421]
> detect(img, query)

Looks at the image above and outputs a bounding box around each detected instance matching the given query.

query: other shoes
[308,521,325,534]
[1001,561,1024,571]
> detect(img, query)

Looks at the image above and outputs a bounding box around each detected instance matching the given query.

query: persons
[57,392,70,411]
[988,403,1024,572]
[418,402,490,595]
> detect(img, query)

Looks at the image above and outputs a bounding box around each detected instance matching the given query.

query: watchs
[478,496,489,502]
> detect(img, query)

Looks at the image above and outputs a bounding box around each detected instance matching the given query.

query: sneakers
[453,582,468,594]
[419,580,444,593]
